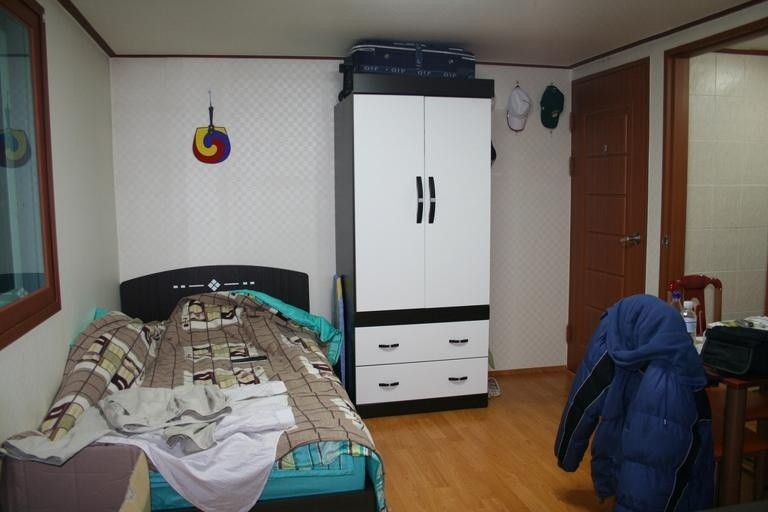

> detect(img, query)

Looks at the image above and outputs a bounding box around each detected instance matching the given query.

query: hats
[506,86,531,133]
[540,84,565,130]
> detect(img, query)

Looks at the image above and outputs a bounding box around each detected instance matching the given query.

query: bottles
[670,291,698,345]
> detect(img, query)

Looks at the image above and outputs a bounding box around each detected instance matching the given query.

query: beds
[110,262,379,512]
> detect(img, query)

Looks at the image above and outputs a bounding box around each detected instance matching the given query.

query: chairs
[599,272,724,510]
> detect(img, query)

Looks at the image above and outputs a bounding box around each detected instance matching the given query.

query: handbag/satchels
[698,325,768,382]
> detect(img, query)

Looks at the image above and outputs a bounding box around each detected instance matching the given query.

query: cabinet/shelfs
[332,71,496,421]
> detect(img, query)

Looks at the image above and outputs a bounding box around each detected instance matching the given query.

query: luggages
[338,38,477,103]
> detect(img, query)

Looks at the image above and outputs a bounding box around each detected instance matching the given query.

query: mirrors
[657,14,767,336]
